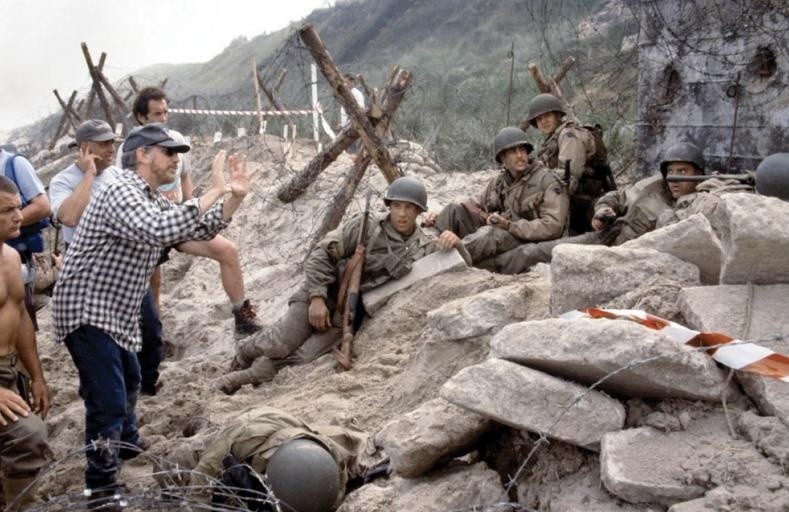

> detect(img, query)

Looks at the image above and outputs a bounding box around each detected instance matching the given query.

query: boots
[232,300,262,339]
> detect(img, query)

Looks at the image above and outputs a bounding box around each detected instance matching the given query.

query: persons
[420,126,570,259]
[1,143,53,376]
[51,120,259,512]
[526,92,617,236]
[48,119,163,397]
[0,172,51,512]
[209,177,472,395]
[115,85,264,340]
[150,403,391,512]
[502,143,705,274]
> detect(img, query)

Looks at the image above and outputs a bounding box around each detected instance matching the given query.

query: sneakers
[209,377,227,395]
[122,435,166,460]
[229,355,247,372]
[183,416,211,437]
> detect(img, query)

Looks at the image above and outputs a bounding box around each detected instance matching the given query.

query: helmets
[383,176,428,212]
[754,153,788,204]
[527,94,566,128]
[495,127,533,163]
[660,142,705,176]
[265,439,342,512]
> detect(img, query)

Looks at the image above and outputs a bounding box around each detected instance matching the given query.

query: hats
[123,125,191,153]
[75,119,117,145]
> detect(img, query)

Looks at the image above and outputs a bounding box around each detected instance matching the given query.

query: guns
[462,197,512,228]
[667,170,755,184]
[332,189,371,374]
